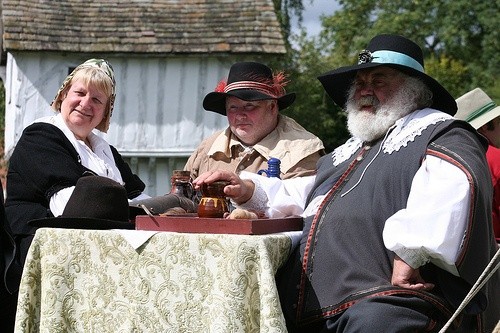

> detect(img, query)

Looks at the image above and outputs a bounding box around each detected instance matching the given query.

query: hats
[317,34,457,117]
[203,62,297,116]
[26,176,154,229]
[51,58,116,133]
[454,88,500,130]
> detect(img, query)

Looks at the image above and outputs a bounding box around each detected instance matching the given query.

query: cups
[195,181,232,219]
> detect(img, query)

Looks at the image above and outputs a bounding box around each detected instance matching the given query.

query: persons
[0,58,198,333]
[455,88,500,246]
[193,34,500,333]
[182,62,326,185]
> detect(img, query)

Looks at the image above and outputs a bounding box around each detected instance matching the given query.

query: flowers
[359,49,379,63]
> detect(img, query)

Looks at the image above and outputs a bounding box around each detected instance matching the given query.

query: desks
[14,227,303,333]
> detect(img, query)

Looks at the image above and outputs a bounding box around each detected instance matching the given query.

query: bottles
[168,170,195,212]
[257,159,282,181]
